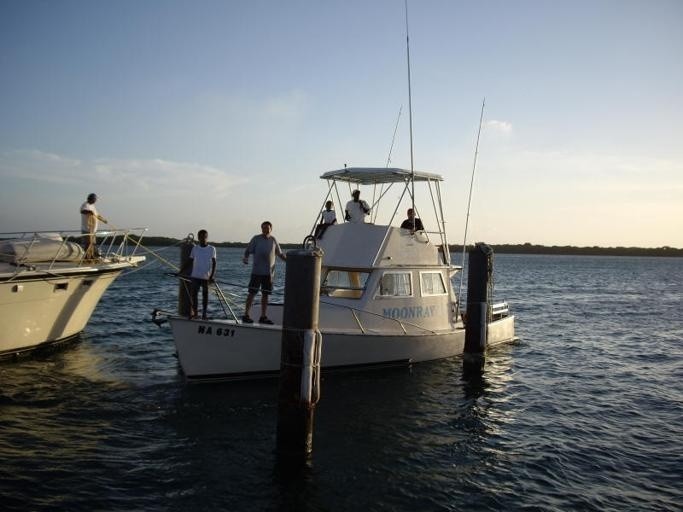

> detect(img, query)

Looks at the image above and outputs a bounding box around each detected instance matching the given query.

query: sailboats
[166,1,522,375]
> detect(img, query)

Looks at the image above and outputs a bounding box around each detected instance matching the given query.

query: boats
[2,225,149,355]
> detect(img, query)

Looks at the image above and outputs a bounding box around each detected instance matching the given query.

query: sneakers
[242,315,253,323]
[258,316,273,324]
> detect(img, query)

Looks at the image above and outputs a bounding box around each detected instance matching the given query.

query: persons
[342,190,370,224]
[174,227,217,322]
[79,191,107,264]
[236,218,287,326]
[306,201,335,241]
[399,208,424,236]
